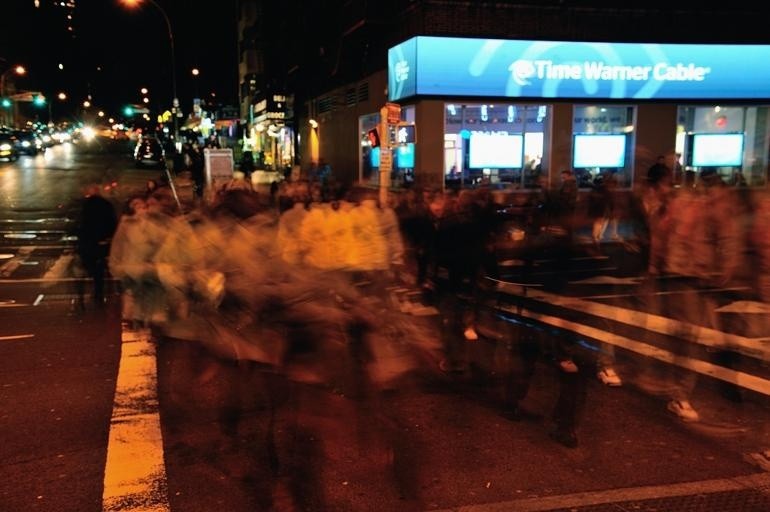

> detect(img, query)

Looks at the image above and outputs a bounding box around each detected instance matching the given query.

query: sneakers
[667,397,700,424]
[464,327,479,341]
[596,366,623,388]
[560,359,578,375]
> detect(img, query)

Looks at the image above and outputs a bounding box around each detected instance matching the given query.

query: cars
[0,126,164,166]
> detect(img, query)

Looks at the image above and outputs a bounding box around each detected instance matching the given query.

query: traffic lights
[368,127,380,148]
[396,122,417,143]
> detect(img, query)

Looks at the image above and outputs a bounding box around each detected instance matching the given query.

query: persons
[62,152,769,512]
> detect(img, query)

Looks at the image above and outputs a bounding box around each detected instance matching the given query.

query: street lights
[47,92,68,122]
[0,64,26,100]
[116,0,182,151]
